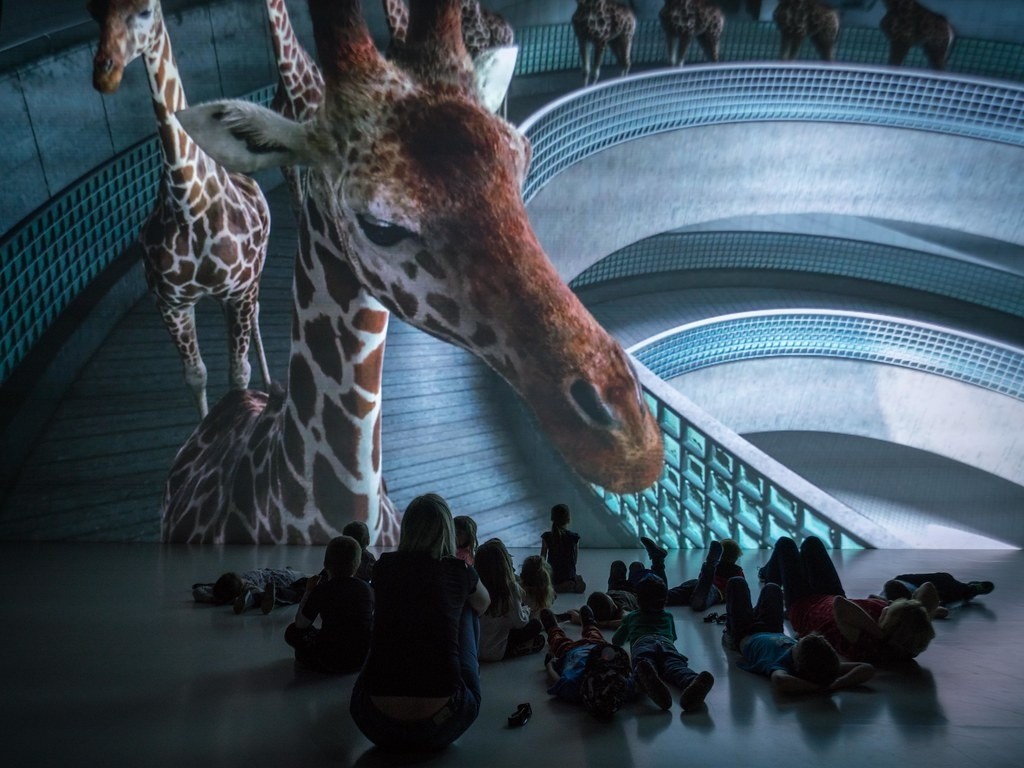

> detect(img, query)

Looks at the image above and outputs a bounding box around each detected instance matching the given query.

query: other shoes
[680,671,714,712]
[641,537,668,560]
[514,634,546,656]
[260,583,275,614]
[723,633,733,644]
[968,581,994,595]
[540,609,558,633]
[706,541,724,566]
[232,589,256,615]
[758,568,768,581]
[635,661,672,709]
[580,605,594,628]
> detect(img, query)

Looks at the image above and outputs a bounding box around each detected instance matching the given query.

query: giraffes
[90,1,962,548]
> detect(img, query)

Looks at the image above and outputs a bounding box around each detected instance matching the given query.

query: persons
[192,566,313,616]
[349,492,492,759]
[758,536,936,663]
[612,573,715,711]
[474,538,545,662]
[879,573,994,619]
[516,555,558,630]
[723,576,875,695]
[453,516,479,566]
[538,605,636,722]
[318,522,375,584]
[284,536,373,675]
[541,504,587,593]
[569,561,646,630]
[640,536,746,612]
